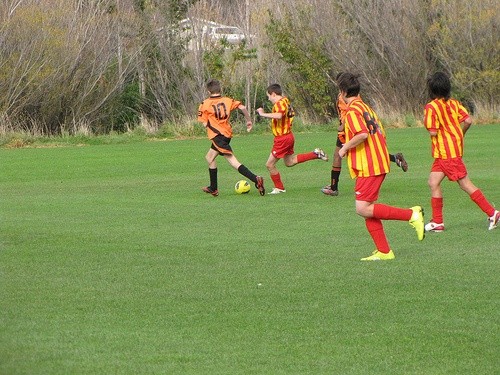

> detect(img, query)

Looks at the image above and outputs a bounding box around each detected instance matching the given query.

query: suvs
[204,26,253,47]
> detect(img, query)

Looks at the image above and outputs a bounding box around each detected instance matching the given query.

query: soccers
[235,179,251,194]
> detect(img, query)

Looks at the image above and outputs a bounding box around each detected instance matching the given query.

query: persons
[423,71,500,233]
[197,79,265,196]
[257,84,329,194]
[321,73,408,196]
[338,73,426,261]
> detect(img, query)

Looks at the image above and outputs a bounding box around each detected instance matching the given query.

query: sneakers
[255,176,265,196]
[409,206,425,240]
[321,185,338,195]
[395,153,408,172]
[313,147,328,162]
[268,188,286,194]
[201,186,219,195]
[425,219,444,233]
[360,250,395,260]
[487,210,500,231]
